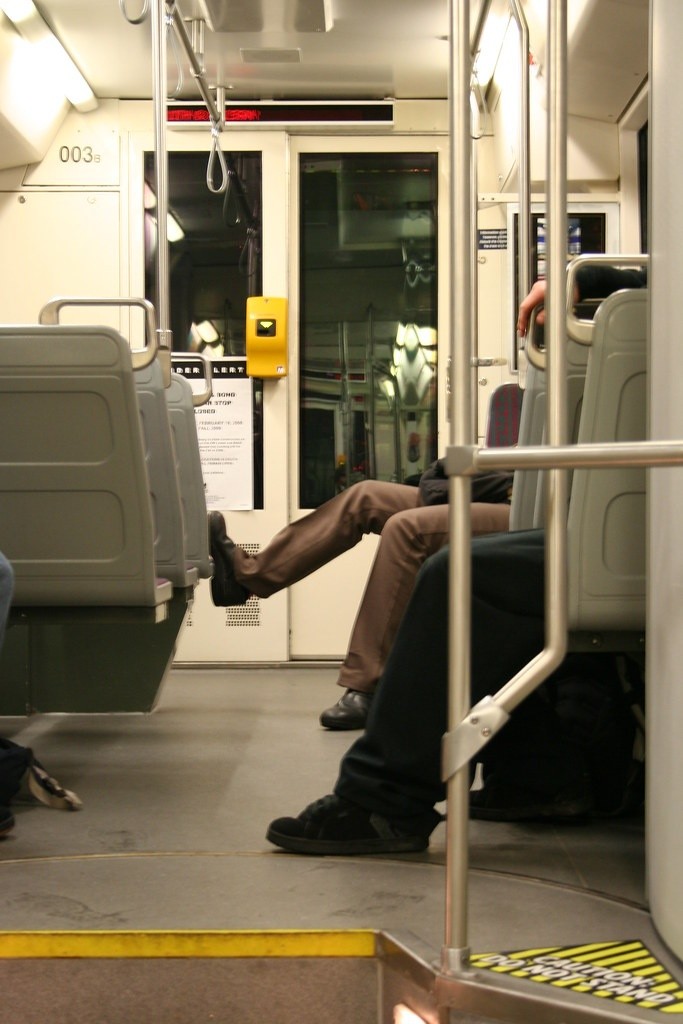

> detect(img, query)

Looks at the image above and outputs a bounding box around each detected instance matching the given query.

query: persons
[201,263,647,855]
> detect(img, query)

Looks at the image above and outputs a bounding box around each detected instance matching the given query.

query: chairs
[0,324,174,625]
[565,253,652,655]
[166,350,215,579]
[39,296,199,589]
[506,298,606,533]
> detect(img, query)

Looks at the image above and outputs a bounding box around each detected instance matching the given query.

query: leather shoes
[318,689,376,729]
[206,511,253,608]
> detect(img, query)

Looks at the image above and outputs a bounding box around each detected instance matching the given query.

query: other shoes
[265,794,430,855]
